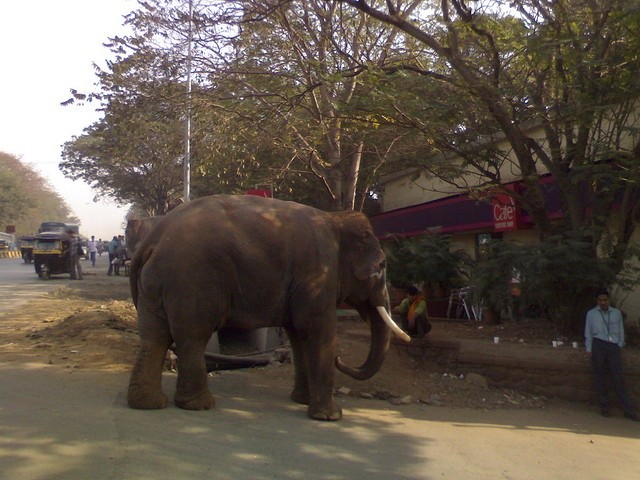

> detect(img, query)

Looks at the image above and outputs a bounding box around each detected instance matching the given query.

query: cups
[572,341,578,348]
[494,336,499,344]
[552,340,557,347]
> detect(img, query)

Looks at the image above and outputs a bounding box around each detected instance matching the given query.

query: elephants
[124,191,415,423]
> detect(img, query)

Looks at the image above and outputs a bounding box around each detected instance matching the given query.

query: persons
[97,238,105,254]
[583,289,640,421]
[118,235,128,252]
[88,236,99,266]
[107,236,120,276]
[390,285,432,345]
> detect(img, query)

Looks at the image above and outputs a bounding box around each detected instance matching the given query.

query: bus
[38,221,79,235]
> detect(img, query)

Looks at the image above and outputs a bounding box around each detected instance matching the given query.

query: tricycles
[20,236,35,263]
[33,231,84,280]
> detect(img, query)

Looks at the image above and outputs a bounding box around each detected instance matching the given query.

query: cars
[0,239,9,251]
